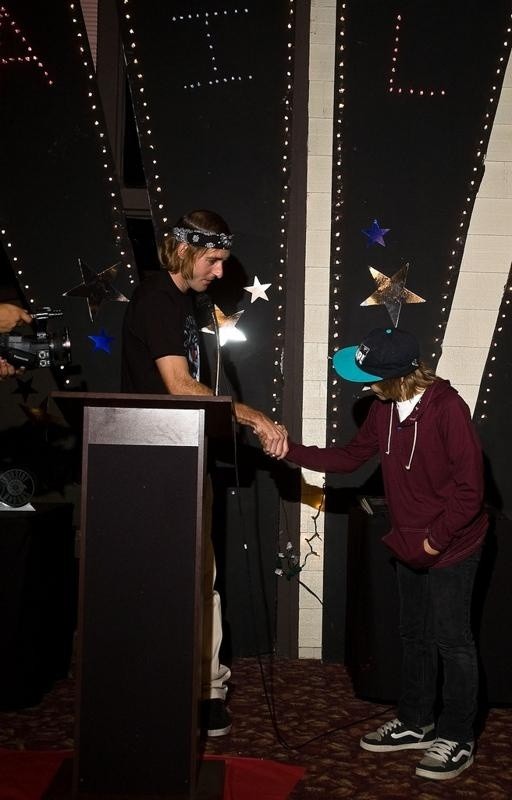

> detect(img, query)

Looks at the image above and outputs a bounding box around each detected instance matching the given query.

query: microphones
[196,292,221,396]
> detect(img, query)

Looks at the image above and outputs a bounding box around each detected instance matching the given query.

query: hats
[333,328,421,383]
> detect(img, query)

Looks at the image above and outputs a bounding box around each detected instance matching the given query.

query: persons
[0,301,35,381]
[121,211,291,740]
[252,329,488,782]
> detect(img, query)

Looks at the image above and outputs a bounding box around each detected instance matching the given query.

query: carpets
[2,748,310,800]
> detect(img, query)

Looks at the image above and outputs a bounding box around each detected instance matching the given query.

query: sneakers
[204,697,233,737]
[360,717,438,752]
[417,737,478,780]
